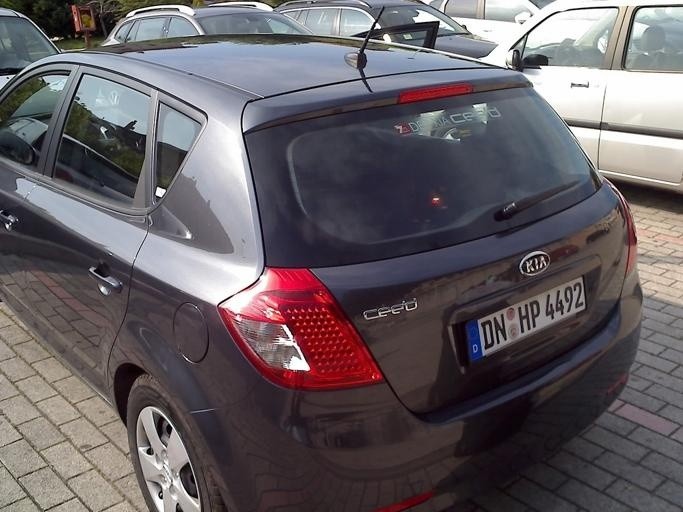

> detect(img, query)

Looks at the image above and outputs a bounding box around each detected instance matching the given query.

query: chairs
[627,25,666,70]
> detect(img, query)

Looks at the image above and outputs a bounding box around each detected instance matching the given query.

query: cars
[0,5,642,512]
